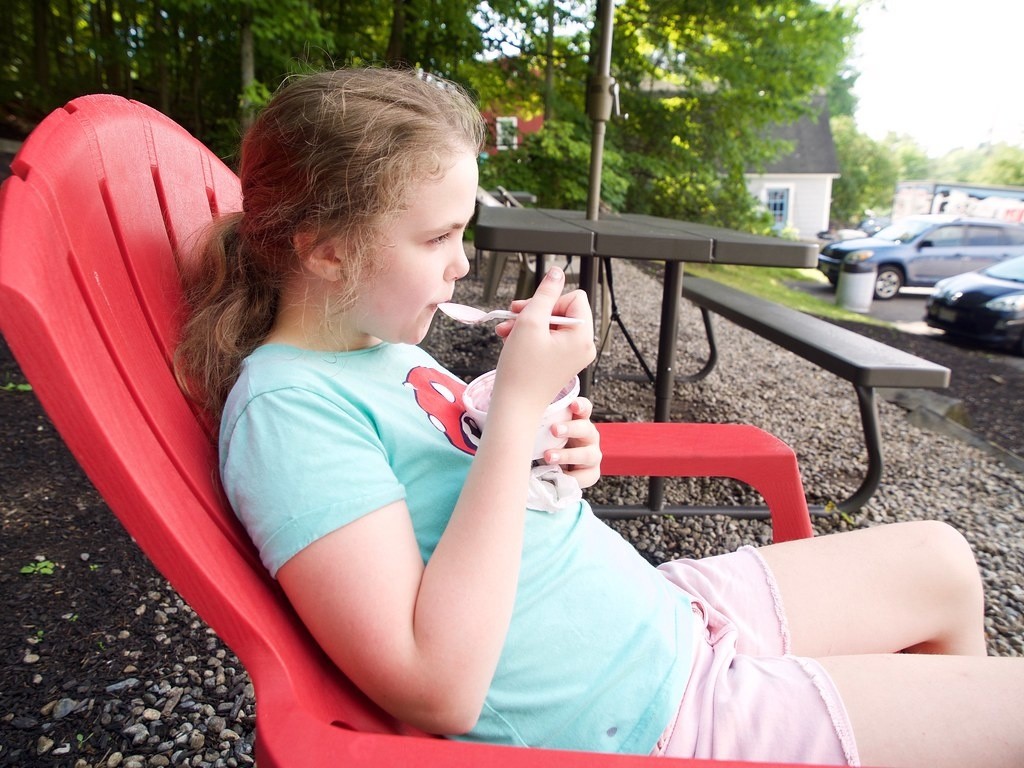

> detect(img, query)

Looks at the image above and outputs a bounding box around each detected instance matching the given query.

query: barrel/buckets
[836,260,876,314]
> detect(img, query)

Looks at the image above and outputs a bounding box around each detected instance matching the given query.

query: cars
[828,212,893,243]
[924,250,1024,357]
[817,212,1024,301]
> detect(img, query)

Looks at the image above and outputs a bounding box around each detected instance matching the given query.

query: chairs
[0,94,876,768]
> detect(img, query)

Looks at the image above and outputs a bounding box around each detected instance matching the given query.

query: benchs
[680,276,950,390]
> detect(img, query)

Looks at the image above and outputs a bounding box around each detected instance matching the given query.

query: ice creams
[470,374,571,414]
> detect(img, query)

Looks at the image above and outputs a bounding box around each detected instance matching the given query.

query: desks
[474,206,884,519]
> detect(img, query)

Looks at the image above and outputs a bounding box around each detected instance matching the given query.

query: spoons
[436,303,587,324]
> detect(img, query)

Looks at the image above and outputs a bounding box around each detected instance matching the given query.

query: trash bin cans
[836,262,878,314]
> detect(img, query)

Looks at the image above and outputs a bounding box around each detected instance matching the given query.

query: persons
[178,64,1024,766]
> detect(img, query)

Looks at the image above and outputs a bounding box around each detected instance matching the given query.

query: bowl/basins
[462,368,580,461]
